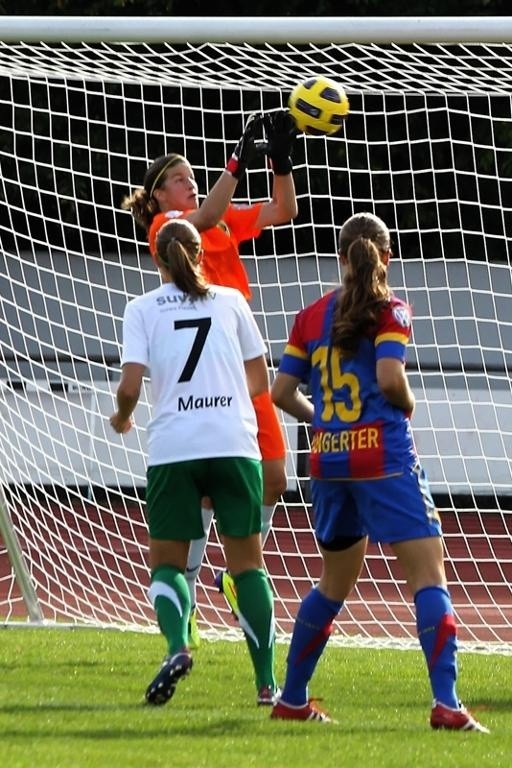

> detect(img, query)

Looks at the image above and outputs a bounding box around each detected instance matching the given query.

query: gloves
[225,112,265,179]
[264,110,300,175]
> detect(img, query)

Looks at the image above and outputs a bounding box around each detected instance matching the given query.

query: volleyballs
[289,77,349,135]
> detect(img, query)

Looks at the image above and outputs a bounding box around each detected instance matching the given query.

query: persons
[109,219,284,707]
[270,212,490,732]
[123,112,300,653]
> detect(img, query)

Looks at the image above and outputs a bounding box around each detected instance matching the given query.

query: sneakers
[145,648,193,705]
[430,698,492,735]
[257,685,337,724]
